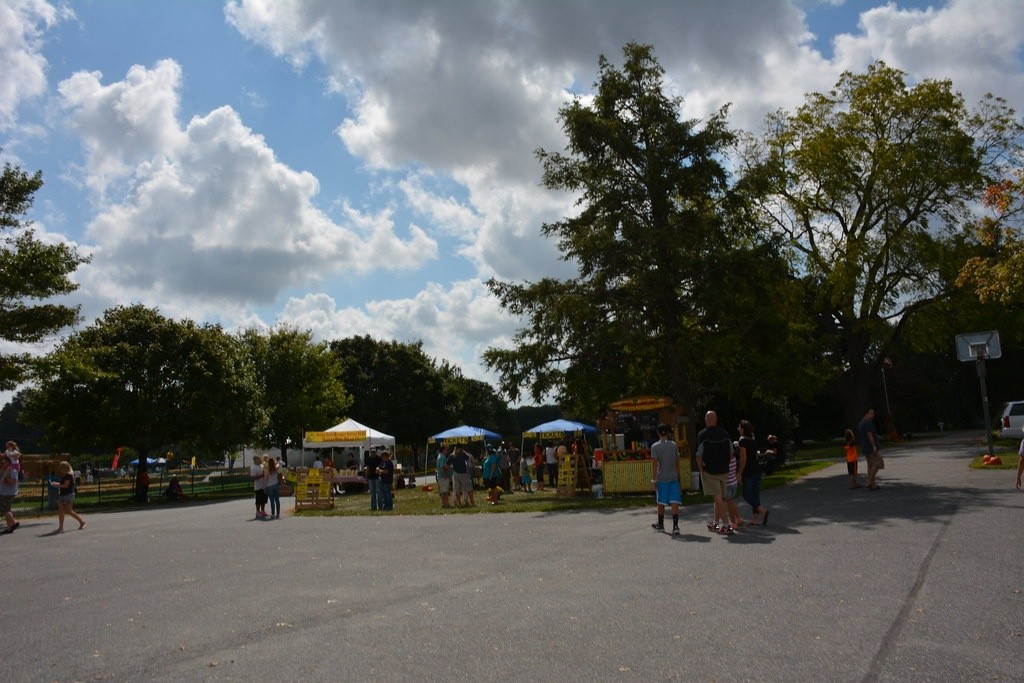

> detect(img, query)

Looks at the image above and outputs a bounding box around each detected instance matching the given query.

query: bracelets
[651,479,656,484]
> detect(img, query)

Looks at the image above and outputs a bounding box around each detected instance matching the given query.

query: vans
[1001,400,1024,439]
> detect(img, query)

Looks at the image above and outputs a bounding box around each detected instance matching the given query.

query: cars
[91,457,224,478]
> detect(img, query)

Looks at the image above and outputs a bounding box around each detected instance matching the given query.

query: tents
[425,425,503,485]
[301,417,396,471]
[519,418,602,473]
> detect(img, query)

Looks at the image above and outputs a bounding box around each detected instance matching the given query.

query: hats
[657,424,669,433]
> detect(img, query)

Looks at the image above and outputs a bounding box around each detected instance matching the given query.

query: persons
[140,469,150,504]
[696,410,769,535]
[0,441,22,533]
[324,457,336,469]
[1015,439,1024,489]
[436,442,521,508]
[844,430,858,489]
[345,453,358,470]
[366,447,394,511]
[650,424,683,536]
[534,437,593,490]
[85,466,93,484]
[114,464,134,478]
[520,454,534,492]
[74,468,82,486]
[313,457,323,469]
[249,454,286,519]
[762,434,785,476]
[50,461,86,532]
[859,407,884,490]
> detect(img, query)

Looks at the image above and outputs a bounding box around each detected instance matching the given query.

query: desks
[332,476,366,483]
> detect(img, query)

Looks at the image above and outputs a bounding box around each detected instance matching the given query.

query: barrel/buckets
[688,472,699,491]
[591,485,605,499]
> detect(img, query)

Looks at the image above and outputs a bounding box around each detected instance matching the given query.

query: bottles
[592,458,596,468]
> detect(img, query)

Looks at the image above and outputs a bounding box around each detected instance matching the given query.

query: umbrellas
[130,457,155,465]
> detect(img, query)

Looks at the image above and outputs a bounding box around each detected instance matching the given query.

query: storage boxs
[297,469,332,501]
[558,455,575,487]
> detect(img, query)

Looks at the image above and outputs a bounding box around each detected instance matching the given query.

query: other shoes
[672,525,680,536]
[0,529,13,533]
[652,523,665,532]
[762,510,769,526]
[256,512,279,520]
[729,522,739,530]
[706,522,719,531]
[10,522,19,532]
[79,522,85,529]
[718,526,734,535]
[870,486,880,490]
[54,529,64,532]
[737,516,744,525]
[747,523,759,527]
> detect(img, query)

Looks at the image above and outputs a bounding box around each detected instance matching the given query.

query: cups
[598,461,602,467]
